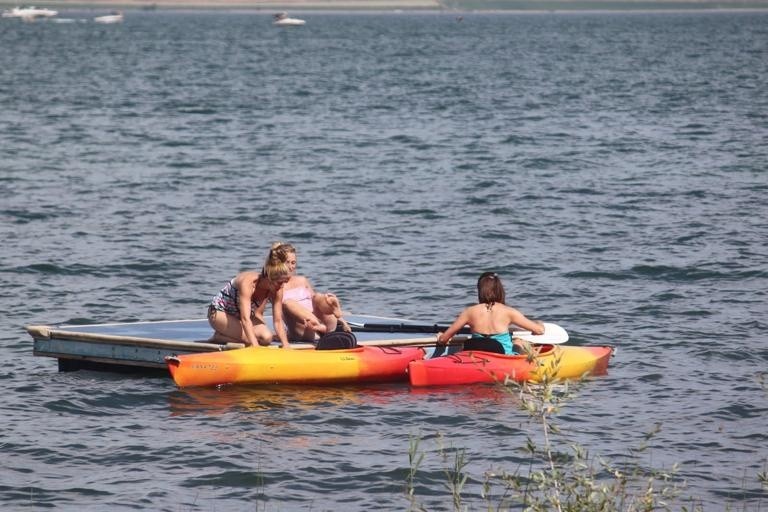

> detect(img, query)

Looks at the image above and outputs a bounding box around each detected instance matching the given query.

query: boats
[164,344,425,389]
[408,344,612,387]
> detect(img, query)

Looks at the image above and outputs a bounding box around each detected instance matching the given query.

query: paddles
[344,321,471,330]
[227,323,568,351]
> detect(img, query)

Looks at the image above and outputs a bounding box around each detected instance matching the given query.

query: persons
[274,243,352,341]
[207,249,290,346]
[434,270,544,355]
